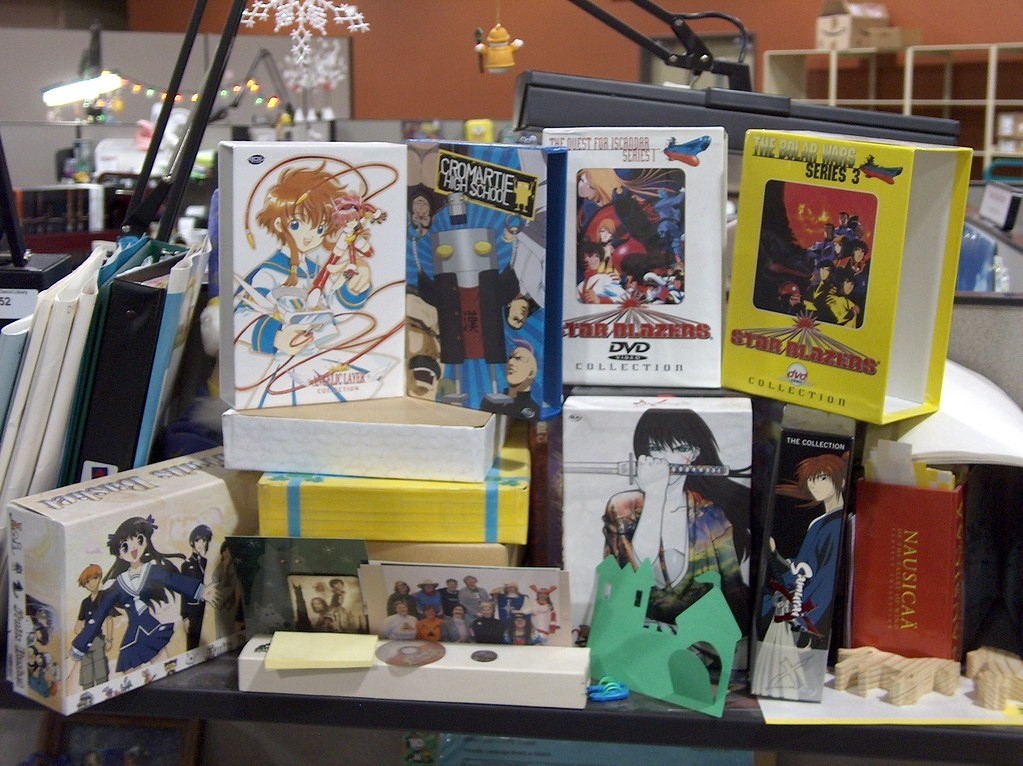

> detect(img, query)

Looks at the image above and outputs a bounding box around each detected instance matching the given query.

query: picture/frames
[34,711,206,766]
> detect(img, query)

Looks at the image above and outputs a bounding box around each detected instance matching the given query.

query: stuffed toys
[474,23,524,74]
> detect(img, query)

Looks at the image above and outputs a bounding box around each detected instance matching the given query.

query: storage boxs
[816,0,900,49]
[6,127,973,717]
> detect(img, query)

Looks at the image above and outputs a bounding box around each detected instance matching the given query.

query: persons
[311,576,560,648]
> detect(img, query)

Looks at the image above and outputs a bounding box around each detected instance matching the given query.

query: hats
[490,586,504,595]
[417,580,439,589]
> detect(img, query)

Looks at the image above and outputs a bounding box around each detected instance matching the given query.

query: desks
[0,636,1023,766]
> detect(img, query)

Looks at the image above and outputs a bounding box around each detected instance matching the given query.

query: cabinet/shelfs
[763,42,1023,185]
